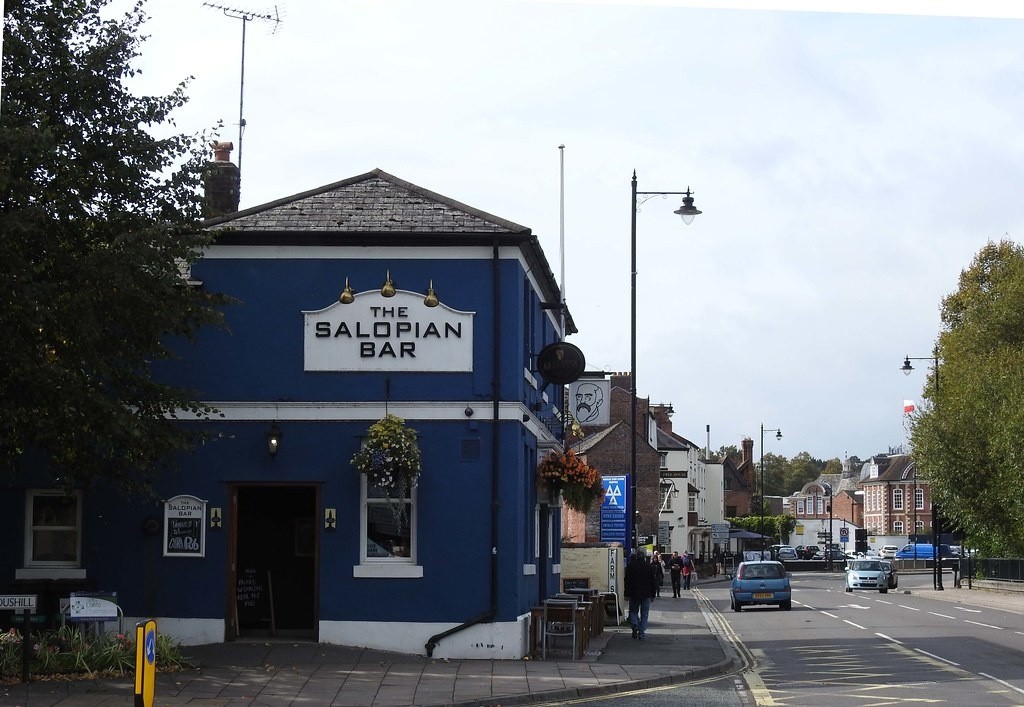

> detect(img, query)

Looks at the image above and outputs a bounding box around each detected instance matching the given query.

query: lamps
[660,479,679,498]
[380,269,397,297]
[540,409,585,441]
[267,419,282,461]
[424,278,440,307]
[340,276,356,304]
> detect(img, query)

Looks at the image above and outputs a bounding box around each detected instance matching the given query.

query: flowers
[534,448,606,513]
[30,624,94,675]
[349,413,423,538]
[85,631,137,678]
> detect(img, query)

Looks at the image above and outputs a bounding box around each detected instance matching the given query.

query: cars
[795,545,819,560]
[726,561,793,613]
[845,559,888,594]
[950,546,961,558]
[840,550,883,561]
[812,549,854,560]
[877,545,900,560]
[767,545,791,558]
[880,560,898,589]
[779,548,797,560]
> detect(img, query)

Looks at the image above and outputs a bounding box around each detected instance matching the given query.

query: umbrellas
[729,530,774,550]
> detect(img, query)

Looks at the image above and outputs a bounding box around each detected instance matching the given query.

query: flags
[904,400,915,413]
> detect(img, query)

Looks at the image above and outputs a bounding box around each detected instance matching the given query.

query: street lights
[822,487,834,571]
[900,343,944,590]
[760,424,783,561]
[631,170,702,562]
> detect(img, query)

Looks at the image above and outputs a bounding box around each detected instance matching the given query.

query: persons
[681,550,696,590]
[646,550,665,603]
[667,551,684,598]
[624,546,657,641]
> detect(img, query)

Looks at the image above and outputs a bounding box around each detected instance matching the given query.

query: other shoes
[637,633,647,639]
[687,585,690,590]
[632,626,638,640]
[678,592,681,598]
[672,594,677,598]
[683,582,687,590]
[657,592,660,598]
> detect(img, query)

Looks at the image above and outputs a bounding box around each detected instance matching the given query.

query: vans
[939,544,951,558]
[823,542,844,551]
[895,544,939,560]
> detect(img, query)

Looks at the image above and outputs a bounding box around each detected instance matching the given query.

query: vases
[371,448,391,473]
[34,650,71,674]
[117,656,135,671]
[548,475,561,490]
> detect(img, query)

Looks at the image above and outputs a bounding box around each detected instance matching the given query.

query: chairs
[542,589,598,660]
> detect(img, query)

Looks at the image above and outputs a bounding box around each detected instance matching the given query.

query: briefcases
[691,571,698,585]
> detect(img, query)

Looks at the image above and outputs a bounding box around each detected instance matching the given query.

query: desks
[587,595,600,638]
[530,604,586,660]
[596,594,605,633]
[574,601,593,649]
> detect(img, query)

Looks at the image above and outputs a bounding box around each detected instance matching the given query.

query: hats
[636,547,646,558]
[683,551,689,557]
